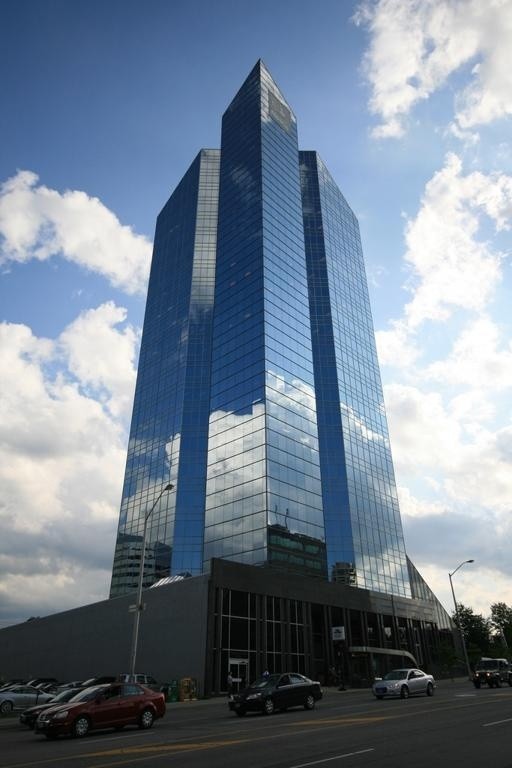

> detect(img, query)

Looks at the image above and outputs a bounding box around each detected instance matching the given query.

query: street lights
[127,481,178,672]
[448,558,478,682]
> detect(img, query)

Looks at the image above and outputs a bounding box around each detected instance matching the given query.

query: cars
[0,673,168,739]
[230,672,325,718]
[370,666,438,701]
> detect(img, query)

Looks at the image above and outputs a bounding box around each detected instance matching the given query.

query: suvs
[473,654,512,688]
